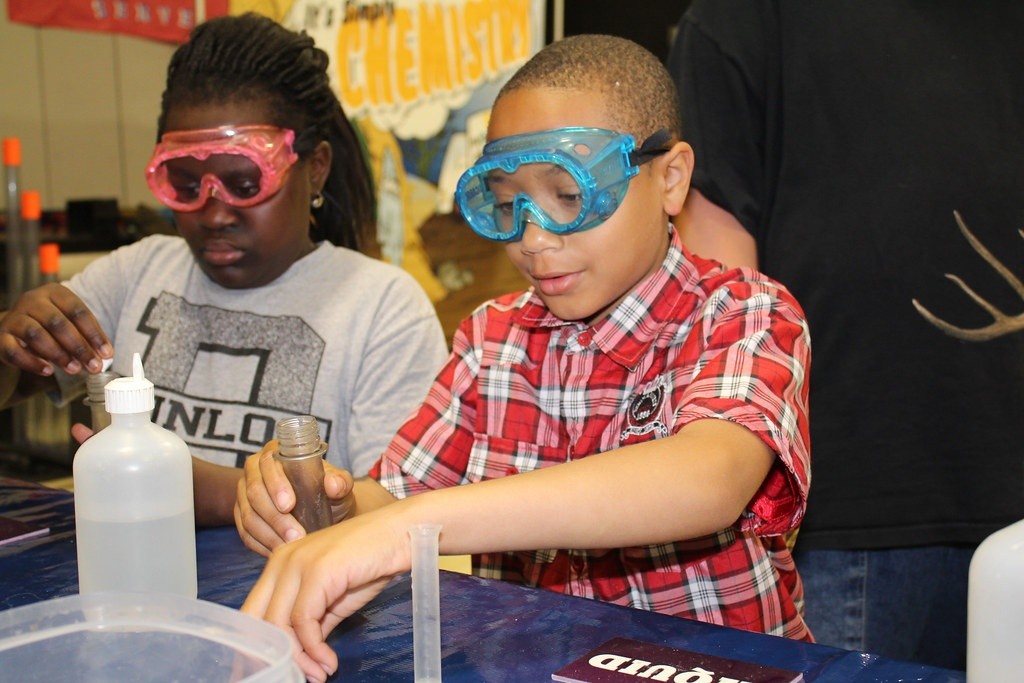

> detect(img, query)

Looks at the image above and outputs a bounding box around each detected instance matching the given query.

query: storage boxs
[0,589,309,681]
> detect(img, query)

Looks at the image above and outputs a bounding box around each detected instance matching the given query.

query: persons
[235,36,816,683]
[0,11,450,525]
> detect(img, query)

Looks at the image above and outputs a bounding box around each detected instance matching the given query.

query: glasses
[145,125,298,212]
[455,127,639,242]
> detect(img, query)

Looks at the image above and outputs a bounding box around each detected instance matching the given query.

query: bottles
[71,370,199,633]
[272,413,334,536]
[0,136,64,451]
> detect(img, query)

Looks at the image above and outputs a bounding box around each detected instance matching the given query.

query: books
[551,636,803,683]
[0,516,49,544]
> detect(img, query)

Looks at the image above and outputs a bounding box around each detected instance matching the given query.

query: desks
[0,482,967,681]
